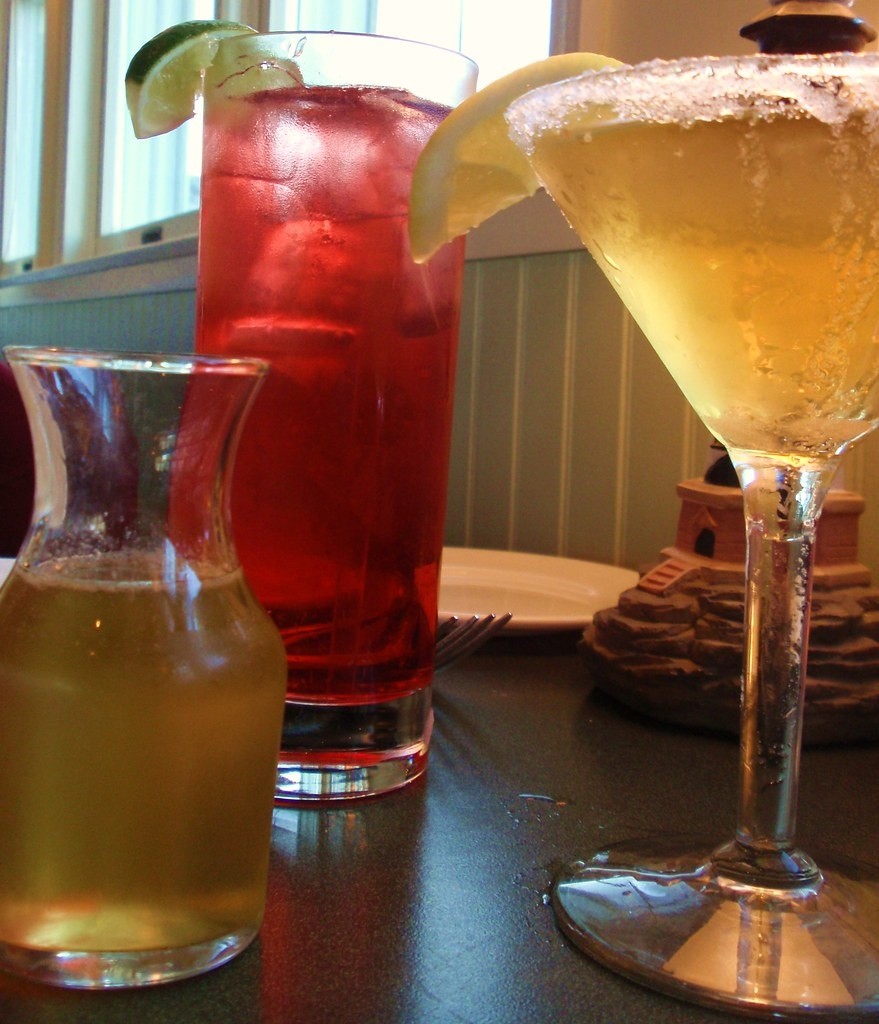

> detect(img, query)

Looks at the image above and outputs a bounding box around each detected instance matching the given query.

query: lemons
[125,19,305,139]
[406,51,634,264]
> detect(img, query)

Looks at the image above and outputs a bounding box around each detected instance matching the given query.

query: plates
[435,547,643,640]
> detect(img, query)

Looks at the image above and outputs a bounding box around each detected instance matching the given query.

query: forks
[434,612,512,677]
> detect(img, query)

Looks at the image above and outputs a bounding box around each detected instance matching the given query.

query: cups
[171,32,480,803]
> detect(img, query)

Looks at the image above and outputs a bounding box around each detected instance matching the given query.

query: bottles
[1,341,289,991]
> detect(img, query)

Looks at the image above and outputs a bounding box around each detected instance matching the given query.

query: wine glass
[501,55,879,1022]
